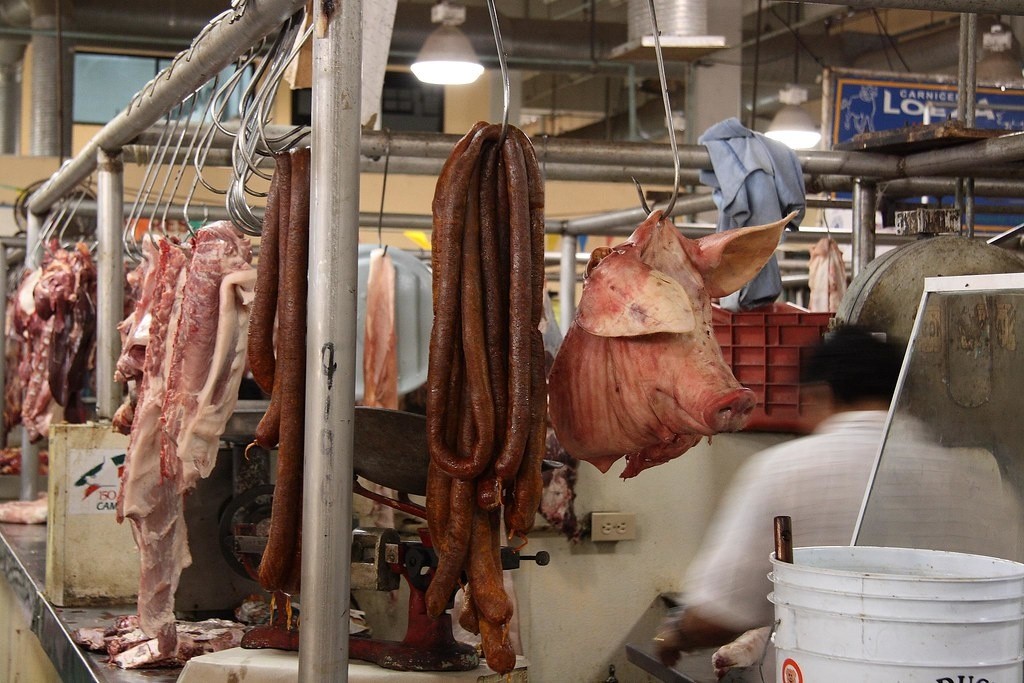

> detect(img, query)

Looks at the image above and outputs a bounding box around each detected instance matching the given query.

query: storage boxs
[709,307,835,433]
[43,423,139,608]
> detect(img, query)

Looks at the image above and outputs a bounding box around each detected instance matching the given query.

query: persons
[652,326,1009,670]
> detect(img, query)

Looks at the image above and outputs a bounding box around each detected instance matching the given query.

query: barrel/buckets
[757,545,1024,683]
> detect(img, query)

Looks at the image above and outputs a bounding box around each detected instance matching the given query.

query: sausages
[423,120,546,674]
[247,143,313,596]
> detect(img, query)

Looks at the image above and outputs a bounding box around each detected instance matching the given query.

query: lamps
[761,107,822,150]
[410,0,485,85]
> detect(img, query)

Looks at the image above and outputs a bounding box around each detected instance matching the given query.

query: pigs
[546,207,802,480]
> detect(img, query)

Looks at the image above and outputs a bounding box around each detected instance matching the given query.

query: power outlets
[589,510,637,542]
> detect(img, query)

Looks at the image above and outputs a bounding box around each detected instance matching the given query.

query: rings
[654,637,665,641]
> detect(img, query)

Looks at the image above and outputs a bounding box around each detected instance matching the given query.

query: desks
[0,523,182,683]
